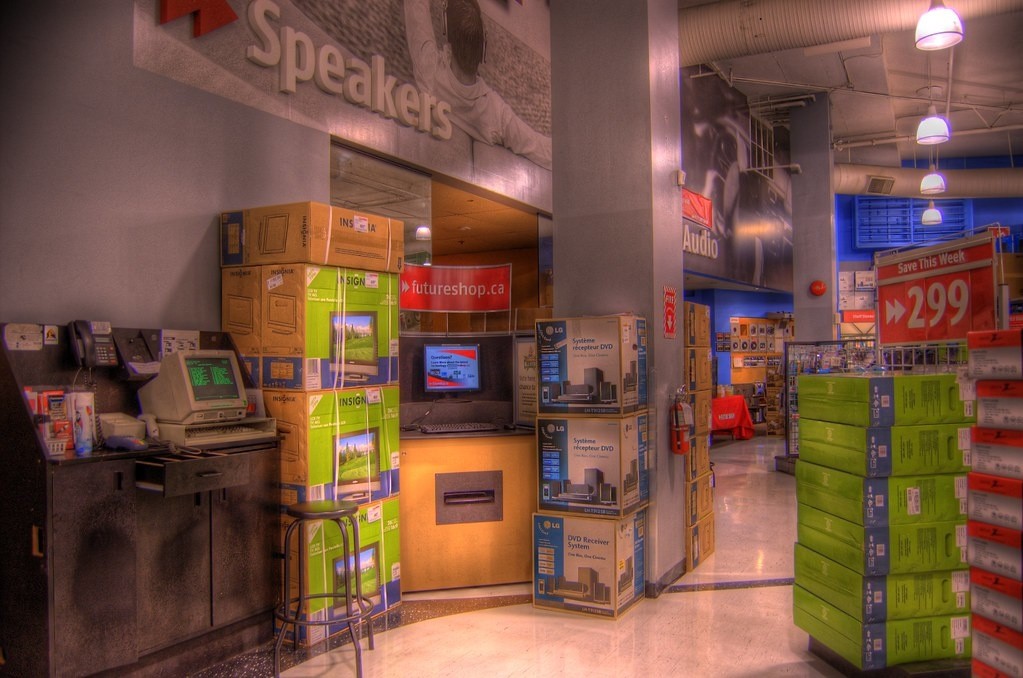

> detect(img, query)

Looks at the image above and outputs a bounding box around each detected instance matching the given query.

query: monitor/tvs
[329,310,379,382]
[332,541,386,617]
[332,423,380,502]
[133,349,248,426]
[422,343,483,403]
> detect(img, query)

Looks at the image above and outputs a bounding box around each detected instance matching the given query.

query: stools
[270,499,375,678]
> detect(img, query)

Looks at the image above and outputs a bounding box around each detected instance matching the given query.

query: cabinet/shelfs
[0,320,286,678]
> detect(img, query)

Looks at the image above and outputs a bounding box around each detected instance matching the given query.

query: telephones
[66,319,118,368]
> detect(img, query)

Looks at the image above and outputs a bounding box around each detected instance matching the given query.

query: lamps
[914,0,964,51]
[922,195,942,225]
[915,51,950,145]
[919,145,946,195]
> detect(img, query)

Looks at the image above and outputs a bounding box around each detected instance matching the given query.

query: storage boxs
[274,495,402,645]
[217,199,406,273]
[261,264,399,392]
[264,385,400,505]
[530,253,1023,678]
[219,266,262,390]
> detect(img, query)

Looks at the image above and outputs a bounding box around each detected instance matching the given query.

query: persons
[403,0,551,172]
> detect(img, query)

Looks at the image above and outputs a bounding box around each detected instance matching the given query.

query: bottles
[717,384,734,397]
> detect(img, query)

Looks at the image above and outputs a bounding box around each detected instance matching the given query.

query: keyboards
[185,424,264,440]
